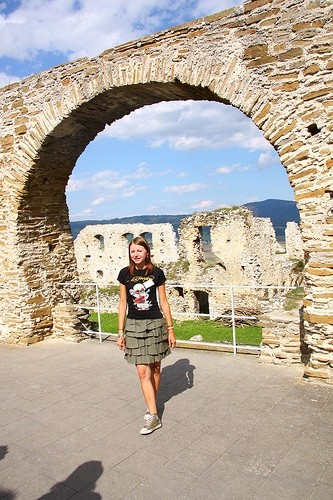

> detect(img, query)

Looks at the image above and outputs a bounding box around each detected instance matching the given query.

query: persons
[116,236,177,436]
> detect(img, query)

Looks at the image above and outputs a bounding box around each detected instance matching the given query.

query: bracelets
[167,327,174,331]
[117,334,124,337]
[117,328,124,330]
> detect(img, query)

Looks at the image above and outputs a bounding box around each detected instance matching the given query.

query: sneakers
[140,413,163,435]
[144,406,155,420]
